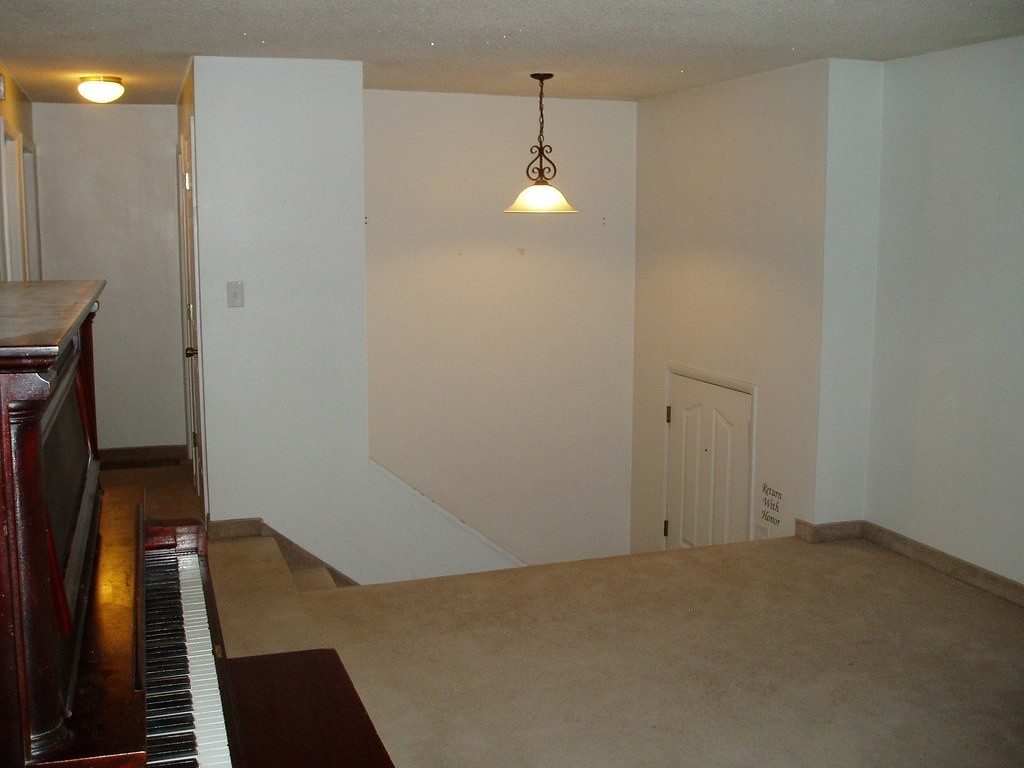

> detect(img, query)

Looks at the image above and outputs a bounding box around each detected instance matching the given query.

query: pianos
[0,279,397,767]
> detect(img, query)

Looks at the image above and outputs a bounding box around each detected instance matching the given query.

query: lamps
[78,76,125,104]
[503,72,579,213]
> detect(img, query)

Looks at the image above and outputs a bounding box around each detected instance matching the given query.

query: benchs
[228,648,396,768]
